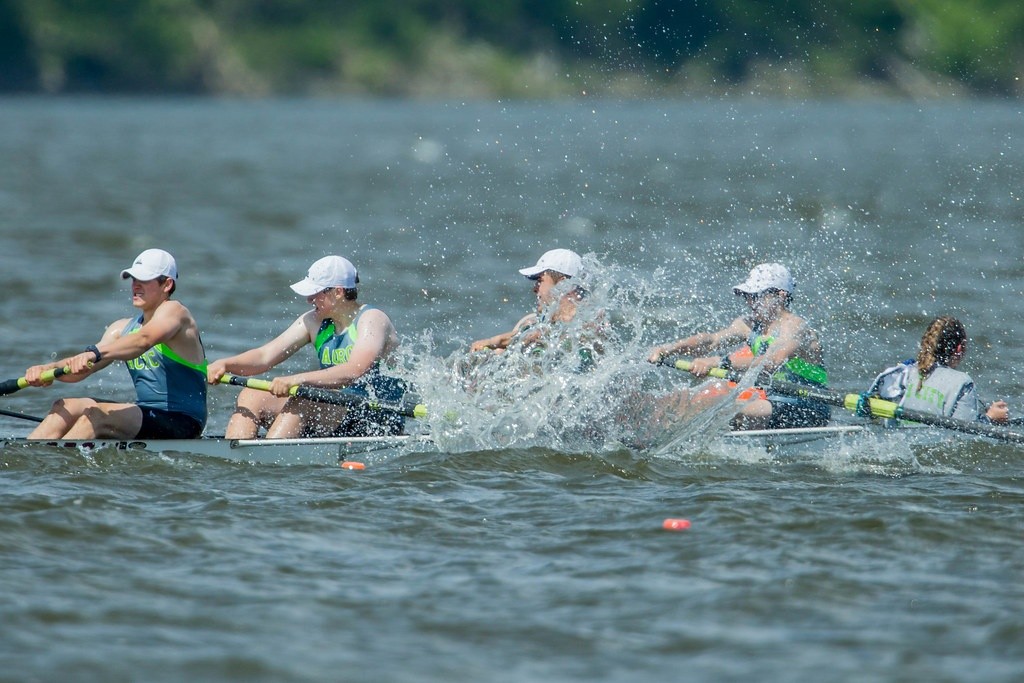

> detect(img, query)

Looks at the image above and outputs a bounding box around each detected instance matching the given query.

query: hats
[519,249,585,282]
[289,256,359,297]
[120,248,178,281]
[732,264,796,302]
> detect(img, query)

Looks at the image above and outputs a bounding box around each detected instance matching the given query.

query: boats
[1,421,1024,474]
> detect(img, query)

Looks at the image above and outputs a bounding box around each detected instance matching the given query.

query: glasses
[743,289,776,302]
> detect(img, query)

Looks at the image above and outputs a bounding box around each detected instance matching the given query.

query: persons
[207,255,406,440]
[872,315,1010,423]
[645,262,830,428]
[24,249,207,441]
[468,248,620,400]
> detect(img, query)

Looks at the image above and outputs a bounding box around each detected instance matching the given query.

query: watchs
[85,345,101,364]
